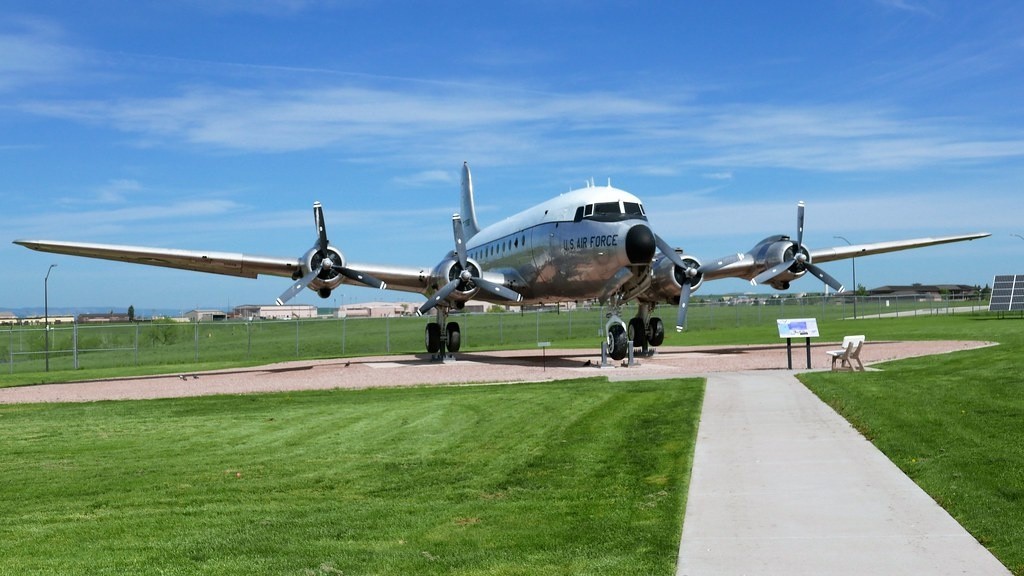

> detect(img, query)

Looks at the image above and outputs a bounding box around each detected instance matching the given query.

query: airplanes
[12,162,994,362]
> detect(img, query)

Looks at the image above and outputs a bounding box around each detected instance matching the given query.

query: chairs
[826,335,866,373]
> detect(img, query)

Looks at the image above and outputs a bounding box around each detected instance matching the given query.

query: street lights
[44,262,57,371]
[833,235,857,319]
